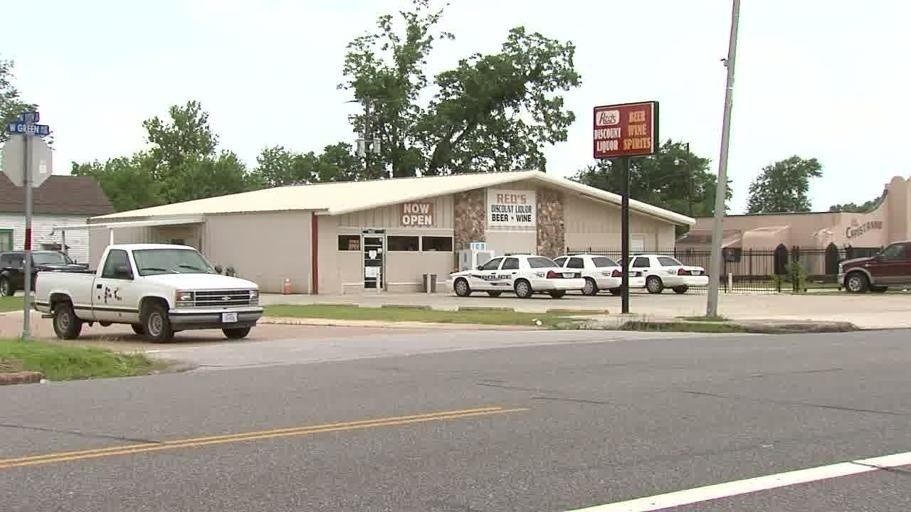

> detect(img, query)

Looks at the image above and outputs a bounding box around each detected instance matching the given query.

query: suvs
[0,248,94,299]
[837,238,911,295]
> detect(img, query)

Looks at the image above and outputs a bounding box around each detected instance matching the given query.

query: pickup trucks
[32,242,268,343]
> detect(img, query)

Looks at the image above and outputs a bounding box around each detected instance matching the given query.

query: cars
[614,252,710,296]
[547,251,622,297]
[444,250,588,300]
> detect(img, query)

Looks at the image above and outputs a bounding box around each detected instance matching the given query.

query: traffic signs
[7,121,51,137]
[18,112,40,123]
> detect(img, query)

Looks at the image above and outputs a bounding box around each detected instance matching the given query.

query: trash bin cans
[423,274,436,293]
